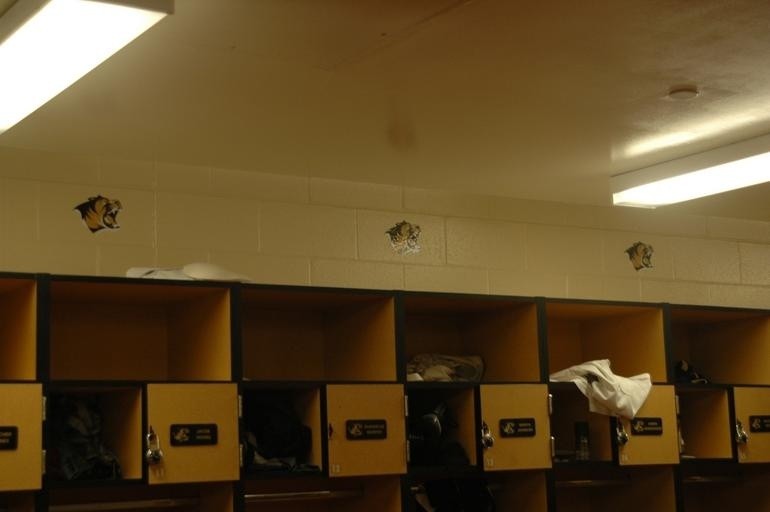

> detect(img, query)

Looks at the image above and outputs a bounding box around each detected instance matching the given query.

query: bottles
[575,421,589,462]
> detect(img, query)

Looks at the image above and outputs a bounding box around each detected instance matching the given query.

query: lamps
[0,0,175,133]
[610,133,770,209]
